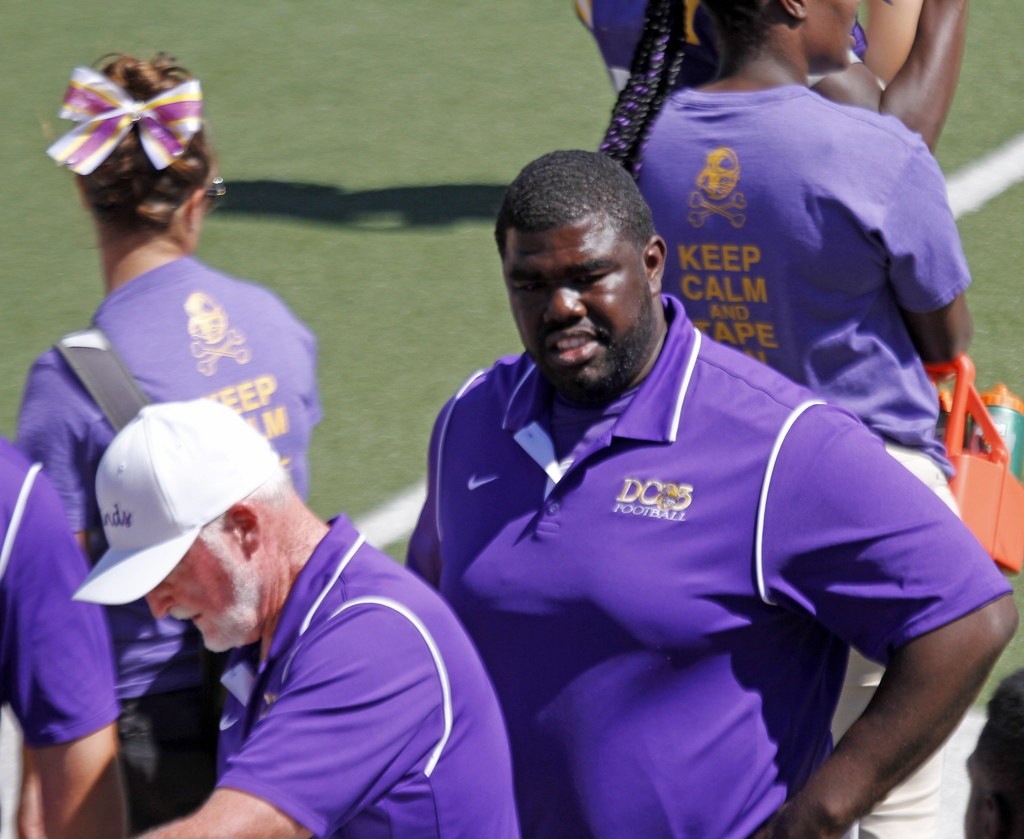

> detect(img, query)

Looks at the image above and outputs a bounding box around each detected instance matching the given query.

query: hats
[70,399,274,603]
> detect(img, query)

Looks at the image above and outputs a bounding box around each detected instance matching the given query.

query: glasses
[201,177,227,216]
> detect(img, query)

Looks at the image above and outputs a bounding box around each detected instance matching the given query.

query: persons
[963,668,1024,839]
[0,437,130,839]
[13,51,325,839]
[403,150,1018,839]
[70,398,520,839]
[597,0,974,839]
[573,0,970,155]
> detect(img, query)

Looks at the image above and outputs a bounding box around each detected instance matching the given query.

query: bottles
[972,381,1023,485]
[932,388,967,449]
